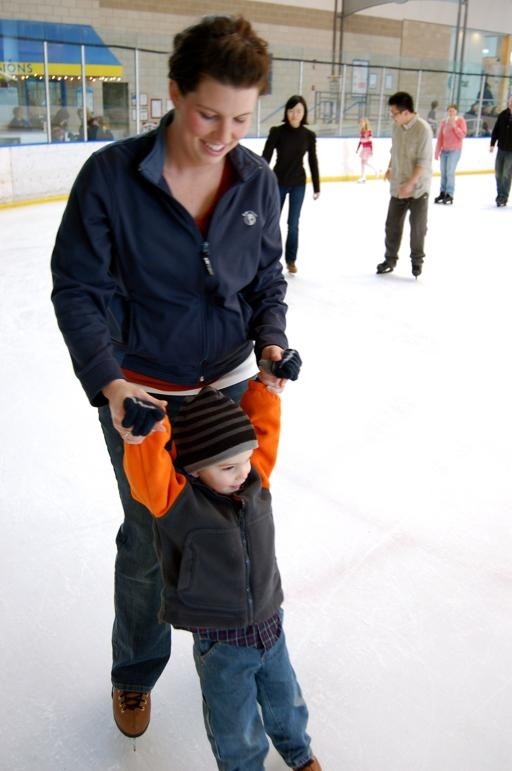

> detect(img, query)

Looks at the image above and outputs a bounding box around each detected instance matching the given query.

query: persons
[259,95,321,274]
[122,348,322,771]
[376,92,433,276]
[428,82,505,137]
[356,118,381,183]
[9,100,117,141]
[51,16,289,739]
[490,94,512,203]
[434,105,468,205]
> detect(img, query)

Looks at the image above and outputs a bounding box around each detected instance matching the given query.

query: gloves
[122,395,164,436]
[260,348,301,380]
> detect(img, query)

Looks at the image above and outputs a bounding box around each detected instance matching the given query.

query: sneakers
[377,260,392,270]
[112,685,151,737]
[412,264,422,276]
[287,262,297,272]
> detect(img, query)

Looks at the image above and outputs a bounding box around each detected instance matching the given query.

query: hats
[173,385,259,473]
